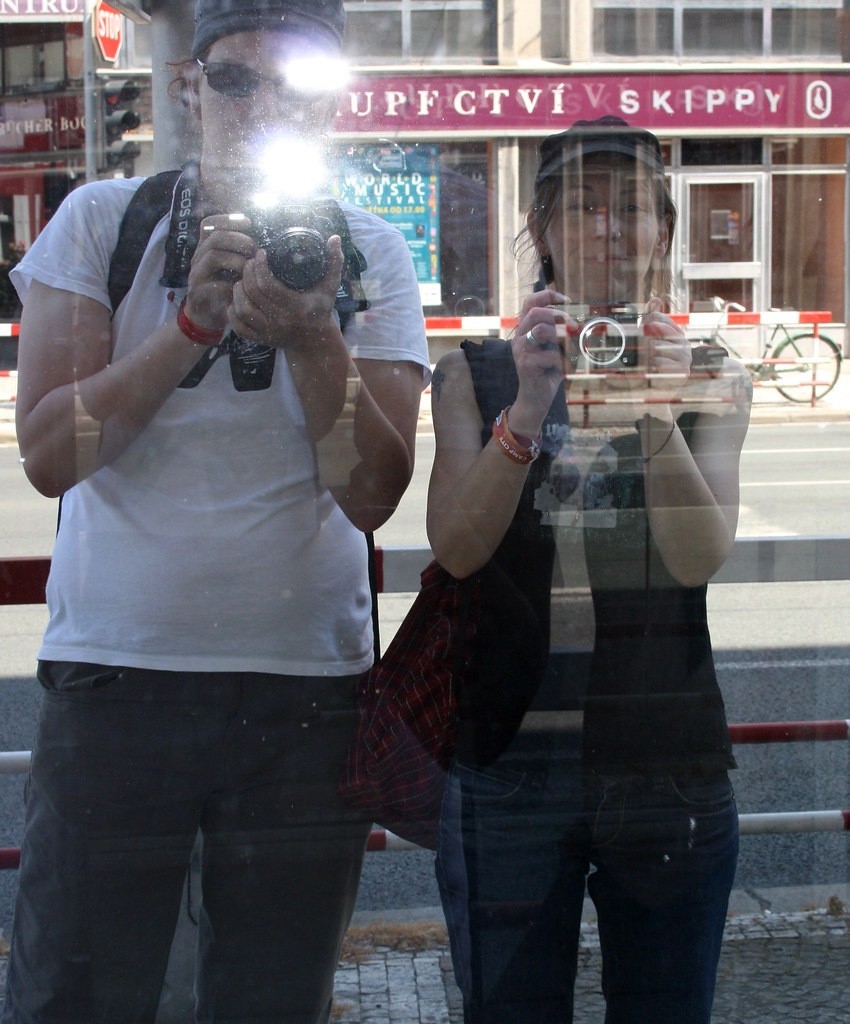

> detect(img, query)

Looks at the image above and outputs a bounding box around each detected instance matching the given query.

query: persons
[426,115,751,1024]
[8,0,433,1024]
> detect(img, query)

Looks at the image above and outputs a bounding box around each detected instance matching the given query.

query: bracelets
[638,417,675,463]
[177,295,225,345]
[492,406,543,464]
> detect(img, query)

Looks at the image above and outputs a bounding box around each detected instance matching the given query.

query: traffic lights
[95,80,142,174]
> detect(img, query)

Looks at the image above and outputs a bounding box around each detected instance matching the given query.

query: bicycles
[699,295,844,410]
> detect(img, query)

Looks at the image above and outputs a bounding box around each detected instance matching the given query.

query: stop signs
[94,0,124,62]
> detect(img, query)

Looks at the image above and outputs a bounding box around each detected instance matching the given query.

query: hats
[193,0,345,62]
[534,114,663,188]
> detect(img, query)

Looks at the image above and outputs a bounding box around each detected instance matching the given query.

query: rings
[527,330,544,348]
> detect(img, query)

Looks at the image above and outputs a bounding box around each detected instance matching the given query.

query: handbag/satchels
[337,558,465,851]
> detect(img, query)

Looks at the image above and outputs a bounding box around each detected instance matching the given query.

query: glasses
[196,58,335,104]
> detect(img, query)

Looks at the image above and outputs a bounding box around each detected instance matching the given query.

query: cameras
[221,198,363,290]
[539,299,651,371]
